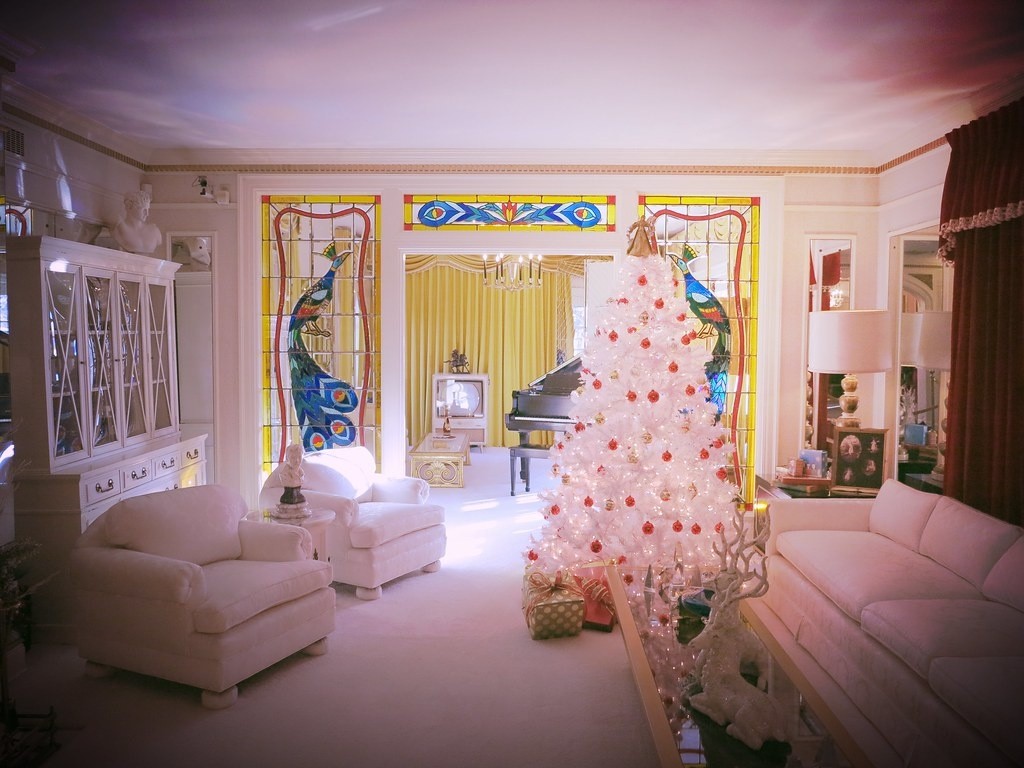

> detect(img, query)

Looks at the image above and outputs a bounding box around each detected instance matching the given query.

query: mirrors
[887,224,955,490]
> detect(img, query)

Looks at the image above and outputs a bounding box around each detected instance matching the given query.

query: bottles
[443,406,451,436]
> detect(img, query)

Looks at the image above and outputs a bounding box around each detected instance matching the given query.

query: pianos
[506,356,586,498]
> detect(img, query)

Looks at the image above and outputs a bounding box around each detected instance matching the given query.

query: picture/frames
[829,426,891,497]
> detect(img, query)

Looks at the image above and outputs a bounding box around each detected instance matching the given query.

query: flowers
[0,537,78,629]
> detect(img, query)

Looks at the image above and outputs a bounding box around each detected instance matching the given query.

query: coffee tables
[597,563,872,767]
[409,431,472,489]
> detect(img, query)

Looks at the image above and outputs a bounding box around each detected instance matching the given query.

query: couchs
[72,484,337,712]
[259,442,451,600]
[742,477,1024,767]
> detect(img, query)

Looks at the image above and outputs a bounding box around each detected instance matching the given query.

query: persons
[110,190,162,253]
[628,216,654,258]
[280,444,305,487]
[452,349,460,363]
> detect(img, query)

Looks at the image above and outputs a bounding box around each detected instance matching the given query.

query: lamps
[807,308,894,430]
[830,288,845,309]
[482,252,544,295]
[902,311,951,446]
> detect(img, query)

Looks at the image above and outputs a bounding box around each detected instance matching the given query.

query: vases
[0,599,24,758]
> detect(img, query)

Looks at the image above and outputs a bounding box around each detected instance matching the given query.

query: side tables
[246,505,336,565]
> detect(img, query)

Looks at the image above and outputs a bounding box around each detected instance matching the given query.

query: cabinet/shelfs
[10,430,211,547]
[432,372,488,455]
[5,233,182,474]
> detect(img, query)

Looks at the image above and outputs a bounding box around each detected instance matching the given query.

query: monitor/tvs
[432,375,488,429]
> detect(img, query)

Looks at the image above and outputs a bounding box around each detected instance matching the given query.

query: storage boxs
[788,458,804,477]
[800,448,828,479]
[905,423,929,446]
[583,579,617,633]
[521,571,585,641]
